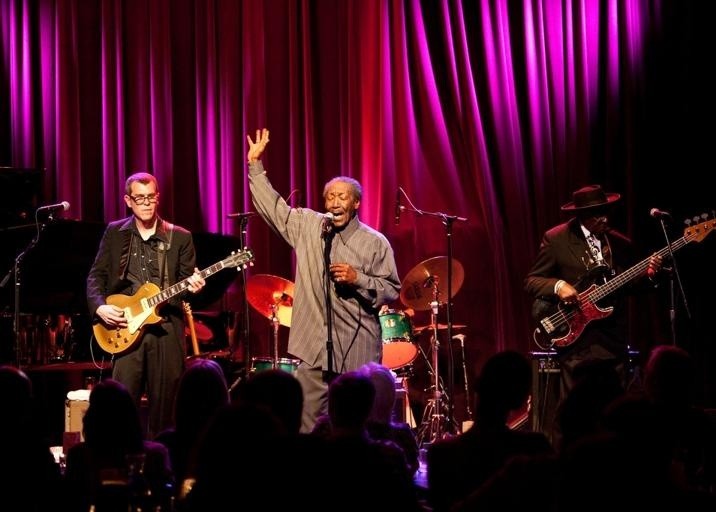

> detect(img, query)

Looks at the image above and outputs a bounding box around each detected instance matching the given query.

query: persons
[87,173,206,439]
[523,184,664,456]
[247,128,402,433]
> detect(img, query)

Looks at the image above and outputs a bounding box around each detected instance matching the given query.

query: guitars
[94,247,255,355]
[531,214,716,346]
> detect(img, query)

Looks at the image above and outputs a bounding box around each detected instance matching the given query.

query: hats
[559,183,622,212]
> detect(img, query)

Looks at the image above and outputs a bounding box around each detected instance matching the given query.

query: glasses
[128,193,161,206]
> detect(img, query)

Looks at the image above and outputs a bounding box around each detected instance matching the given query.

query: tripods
[411,338,460,450]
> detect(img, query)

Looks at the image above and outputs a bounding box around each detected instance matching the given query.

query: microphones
[36,199,73,216]
[226,210,256,223]
[392,187,404,225]
[320,210,336,239]
[648,208,674,221]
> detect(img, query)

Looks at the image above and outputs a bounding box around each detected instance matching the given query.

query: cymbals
[401,255,464,311]
[244,273,295,327]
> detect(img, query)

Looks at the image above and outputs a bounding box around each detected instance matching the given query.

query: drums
[377,309,420,372]
[250,355,303,373]
